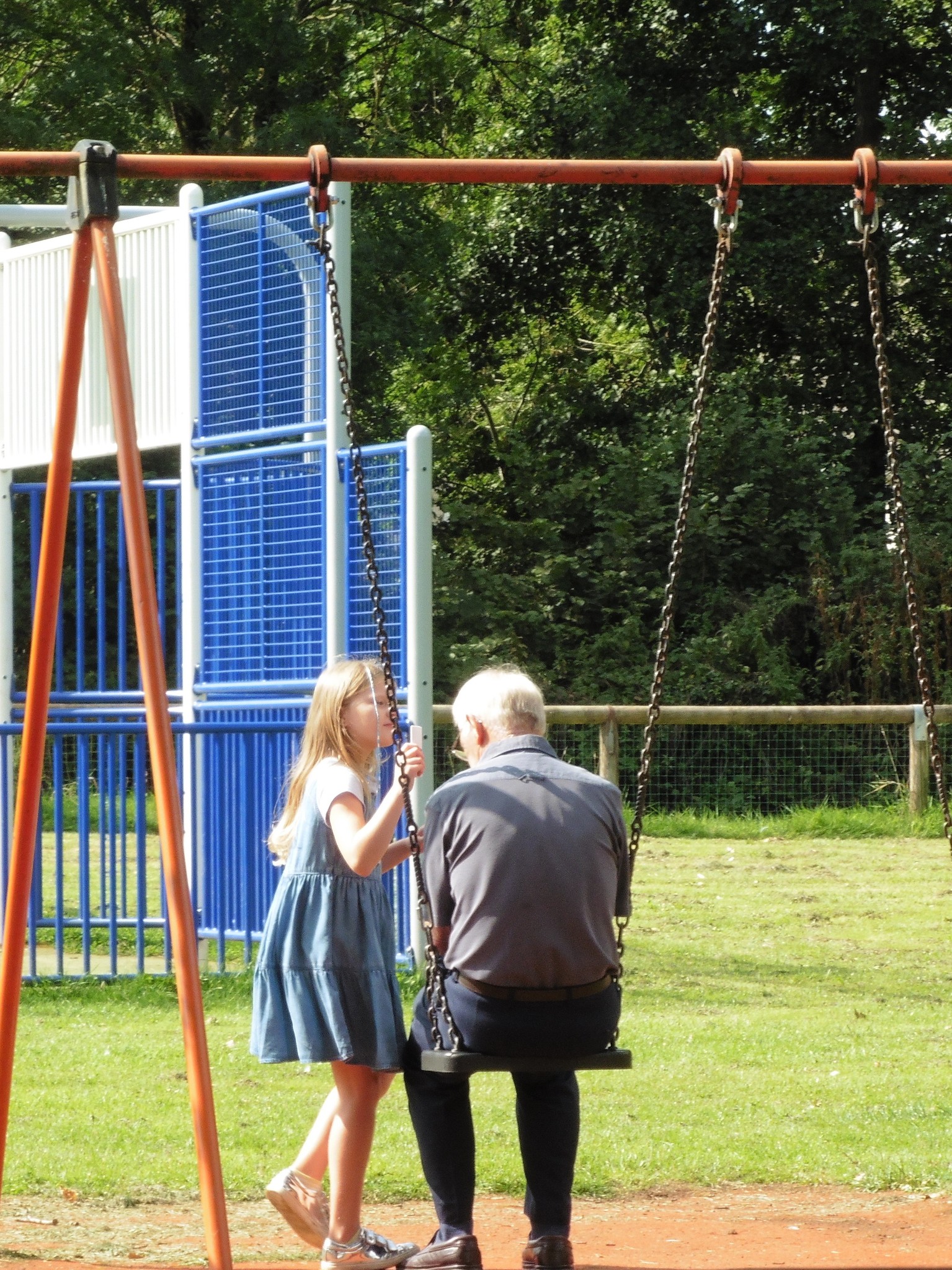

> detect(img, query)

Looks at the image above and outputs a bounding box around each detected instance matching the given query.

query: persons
[395,661,631,1270]
[247,657,420,1270]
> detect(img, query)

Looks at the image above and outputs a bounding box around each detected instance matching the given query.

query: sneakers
[265,1168,332,1248]
[318,1225,418,1270]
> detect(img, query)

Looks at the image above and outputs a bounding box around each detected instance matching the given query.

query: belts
[454,973,613,1003]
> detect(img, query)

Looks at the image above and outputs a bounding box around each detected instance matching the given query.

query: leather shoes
[521,1231,575,1270]
[393,1230,483,1270]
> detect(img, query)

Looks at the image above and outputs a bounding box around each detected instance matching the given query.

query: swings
[849,188,952,863]
[306,183,740,1071]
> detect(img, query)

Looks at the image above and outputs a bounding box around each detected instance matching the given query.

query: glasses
[451,734,469,763]
